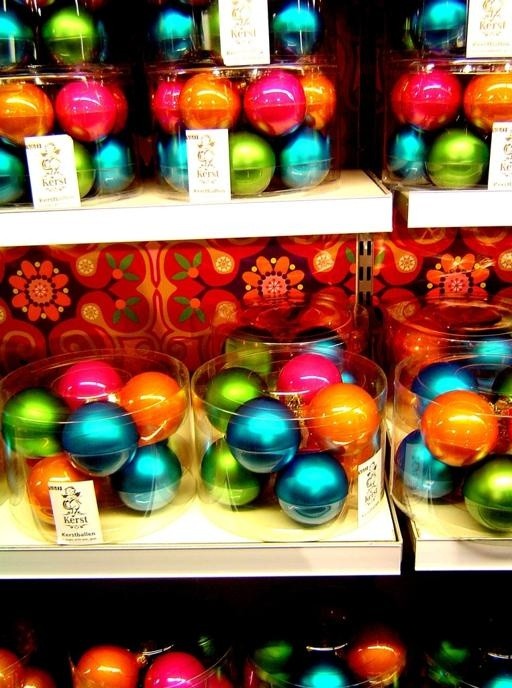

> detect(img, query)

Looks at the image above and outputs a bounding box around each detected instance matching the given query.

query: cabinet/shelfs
[0,0,405,688]
[394,0,512,688]
